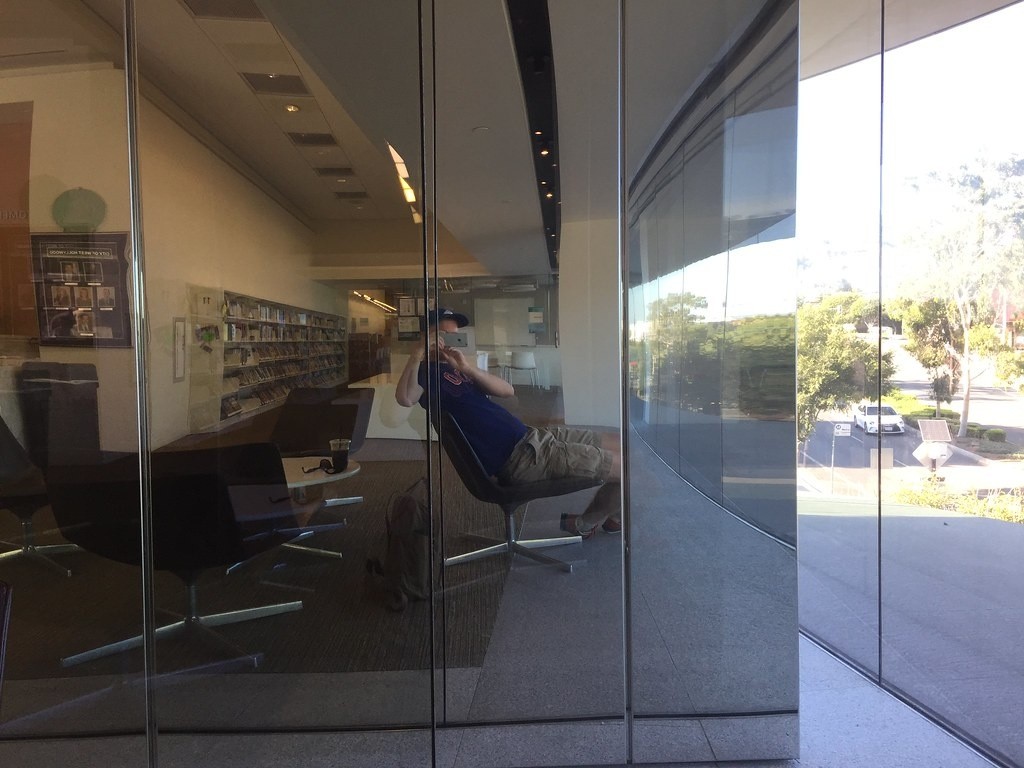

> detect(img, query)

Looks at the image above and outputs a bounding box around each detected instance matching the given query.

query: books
[220,290,346,420]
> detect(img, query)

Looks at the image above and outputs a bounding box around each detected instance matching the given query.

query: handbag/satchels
[385,477,439,601]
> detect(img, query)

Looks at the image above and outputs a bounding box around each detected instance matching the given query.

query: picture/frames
[172,316,187,383]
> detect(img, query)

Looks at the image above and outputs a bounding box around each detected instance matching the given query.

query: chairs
[0,361,375,670]
[431,411,583,572]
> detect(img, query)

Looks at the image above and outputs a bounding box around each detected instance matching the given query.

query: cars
[853,403,905,434]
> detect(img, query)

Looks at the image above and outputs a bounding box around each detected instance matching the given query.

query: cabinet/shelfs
[193,286,350,433]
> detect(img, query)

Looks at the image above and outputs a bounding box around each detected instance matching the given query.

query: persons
[99,289,114,306]
[53,286,69,306]
[63,263,80,281]
[76,288,91,306]
[87,263,101,281]
[77,313,92,333]
[396,305,622,538]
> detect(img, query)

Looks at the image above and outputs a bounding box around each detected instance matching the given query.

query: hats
[420,308,470,329]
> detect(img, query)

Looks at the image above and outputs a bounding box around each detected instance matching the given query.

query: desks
[276,454,363,558]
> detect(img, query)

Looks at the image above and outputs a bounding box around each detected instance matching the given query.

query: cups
[330,439,351,472]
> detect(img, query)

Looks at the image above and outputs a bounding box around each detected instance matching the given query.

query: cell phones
[440,333,470,347]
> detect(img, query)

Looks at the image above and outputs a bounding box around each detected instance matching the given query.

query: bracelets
[410,350,425,362]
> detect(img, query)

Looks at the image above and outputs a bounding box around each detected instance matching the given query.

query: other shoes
[602,518,622,534]
[560,512,598,539]
